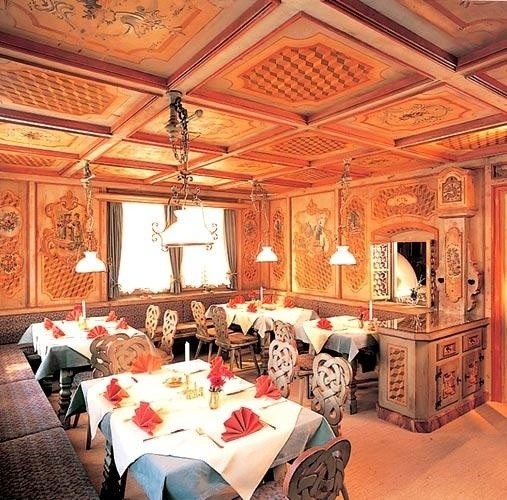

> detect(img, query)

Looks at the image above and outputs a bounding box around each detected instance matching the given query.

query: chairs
[311,352,353,438]
[190,290,379,415]
[17,303,179,450]
[232,437,351,500]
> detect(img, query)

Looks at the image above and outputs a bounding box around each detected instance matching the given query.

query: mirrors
[393,241,426,304]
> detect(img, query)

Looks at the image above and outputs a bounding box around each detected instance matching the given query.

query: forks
[195,427,224,448]
[260,400,288,411]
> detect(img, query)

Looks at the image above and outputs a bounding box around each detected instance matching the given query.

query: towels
[221,406,265,444]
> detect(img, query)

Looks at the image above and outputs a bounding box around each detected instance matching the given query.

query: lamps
[75,160,113,273]
[151,90,218,250]
[329,158,357,266]
[248,179,277,263]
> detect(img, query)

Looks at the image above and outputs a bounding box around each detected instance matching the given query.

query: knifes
[226,385,256,395]
[143,428,186,443]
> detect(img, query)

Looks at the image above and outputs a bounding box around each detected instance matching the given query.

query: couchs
[0,307,110,384]
[0,379,62,443]
[111,294,272,342]
[0,427,100,500]
